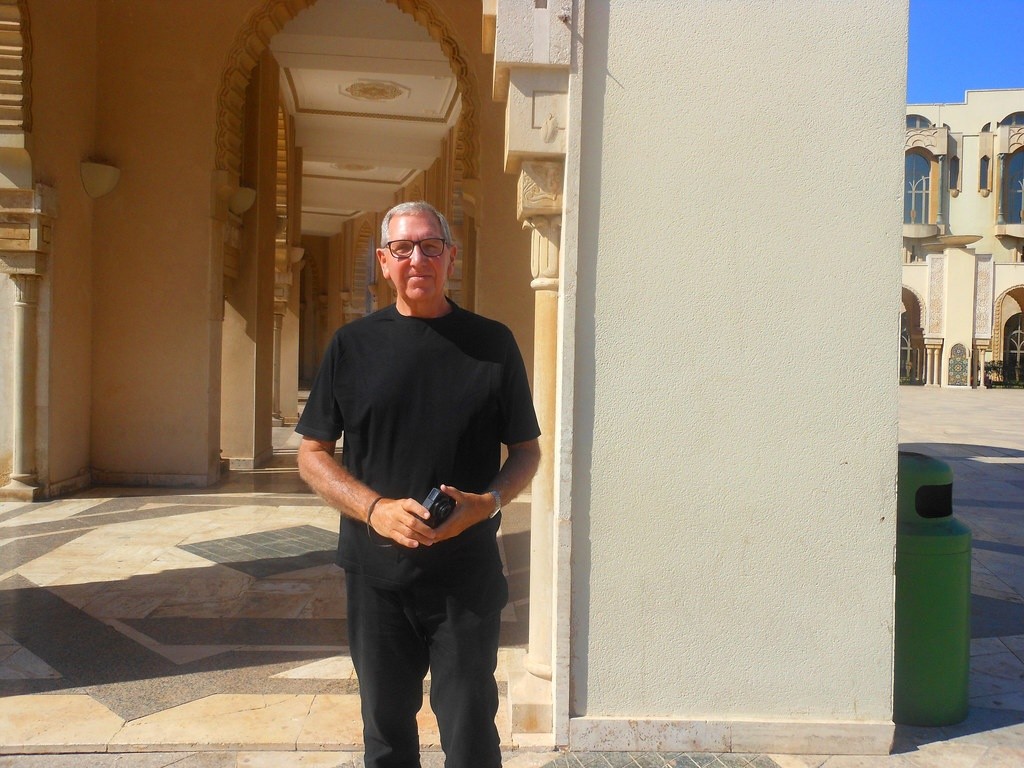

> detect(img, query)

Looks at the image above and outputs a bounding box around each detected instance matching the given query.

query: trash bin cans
[892,451,974,728]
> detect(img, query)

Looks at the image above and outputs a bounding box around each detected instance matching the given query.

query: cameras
[416,488,456,529]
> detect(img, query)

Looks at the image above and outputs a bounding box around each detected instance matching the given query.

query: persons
[293,201,540,768]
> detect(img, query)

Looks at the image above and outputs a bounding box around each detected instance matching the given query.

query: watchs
[488,488,501,519]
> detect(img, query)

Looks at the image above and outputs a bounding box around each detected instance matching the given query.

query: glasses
[382,237,448,258]
